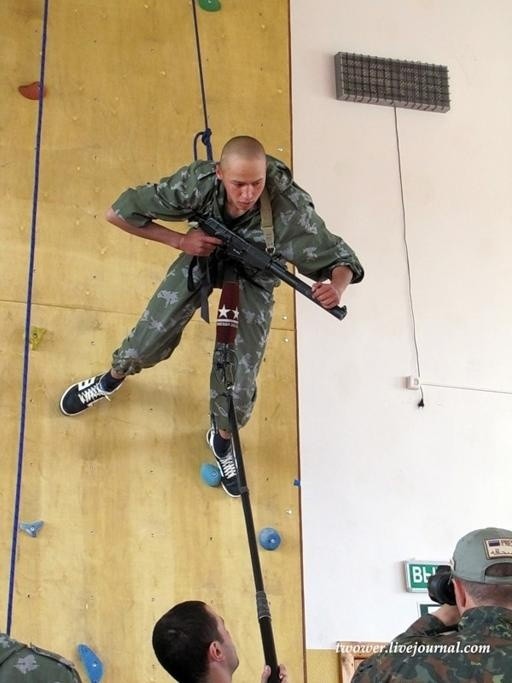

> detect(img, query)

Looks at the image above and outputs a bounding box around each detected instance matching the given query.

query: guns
[188,208,347,320]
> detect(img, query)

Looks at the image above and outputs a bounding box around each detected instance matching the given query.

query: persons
[349,526,512,683]
[152,600,291,683]
[59,135,364,500]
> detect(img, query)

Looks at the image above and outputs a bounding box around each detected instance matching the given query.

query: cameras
[428,565,457,606]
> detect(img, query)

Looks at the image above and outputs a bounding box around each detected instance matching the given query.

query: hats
[442,528,511,585]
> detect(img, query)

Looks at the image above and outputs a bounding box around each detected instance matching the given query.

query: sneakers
[59,373,123,417]
[206,428,241,497]
